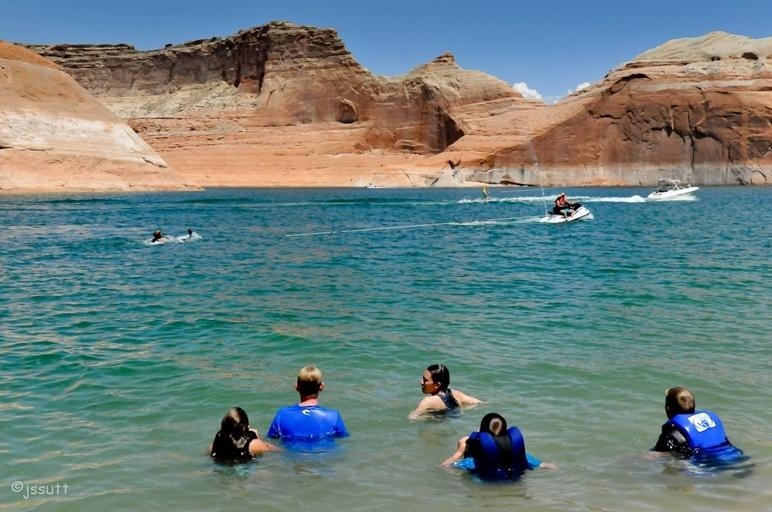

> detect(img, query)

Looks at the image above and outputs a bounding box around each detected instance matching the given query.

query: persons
[152,228,200,246]
[206,365,351,478]
[553,192,575,220]
[408,364,480,430]
[481,185,491,200]
[644,387,753,482]
[436,412,554,481]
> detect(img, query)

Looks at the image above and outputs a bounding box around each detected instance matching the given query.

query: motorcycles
[540,201,591,224]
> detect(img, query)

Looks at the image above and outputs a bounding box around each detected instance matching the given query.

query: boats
[647,177,700,201]
